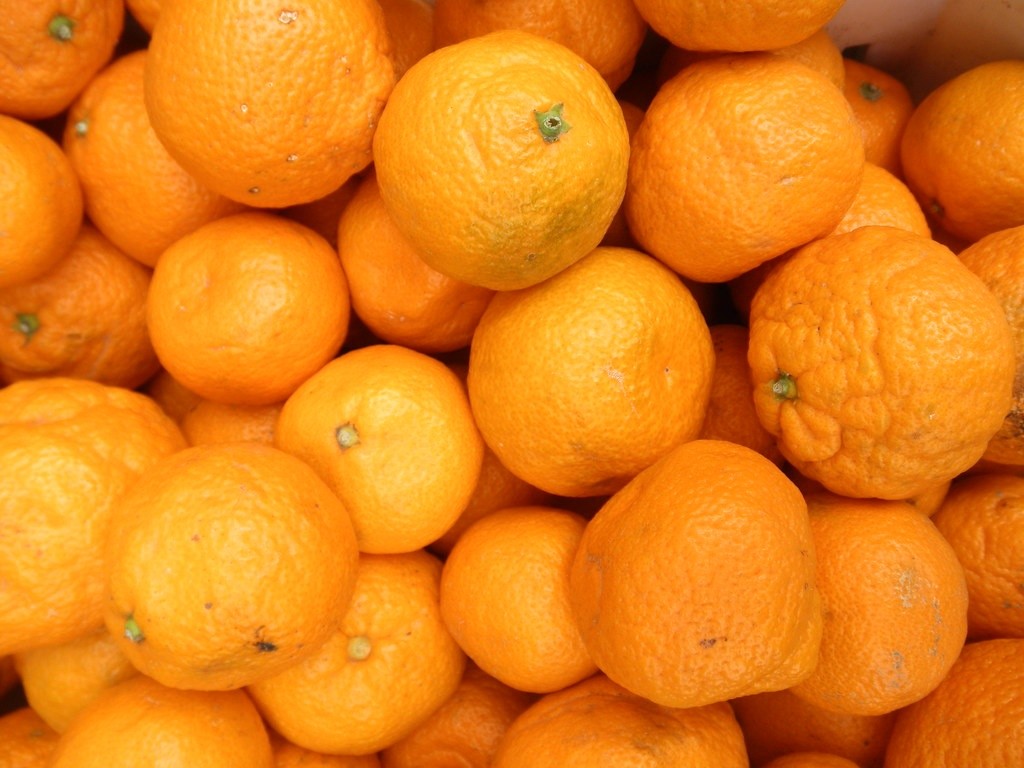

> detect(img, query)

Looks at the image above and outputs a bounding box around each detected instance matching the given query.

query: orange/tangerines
[0,0,1024,768]
